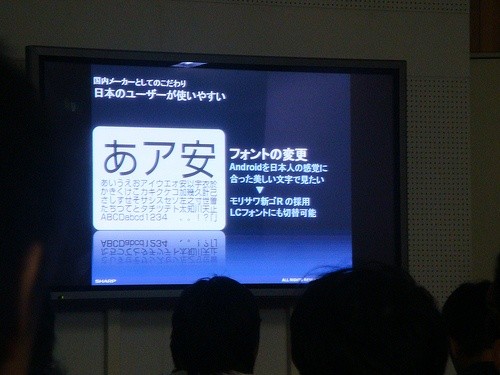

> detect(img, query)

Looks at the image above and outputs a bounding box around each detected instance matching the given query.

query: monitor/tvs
[26,46,408,312]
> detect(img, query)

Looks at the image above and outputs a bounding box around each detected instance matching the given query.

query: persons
[171,276,261,375]
[289,262,449,375]
[444,280,500,375]
[0,39,54,375]
